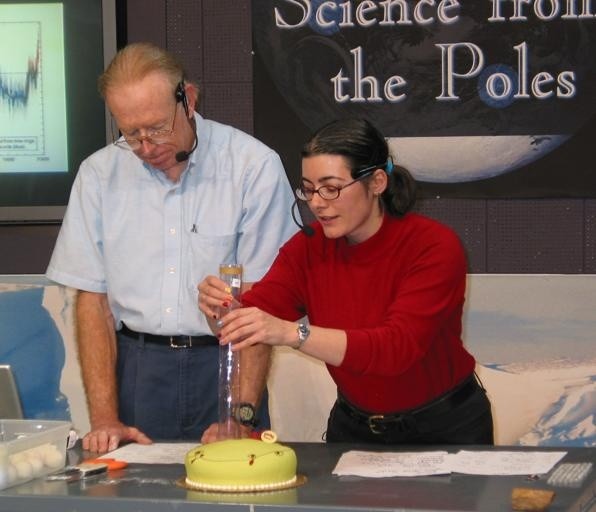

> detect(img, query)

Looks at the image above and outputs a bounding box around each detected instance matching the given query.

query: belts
[121,327,219,353]
[339,375,479,437]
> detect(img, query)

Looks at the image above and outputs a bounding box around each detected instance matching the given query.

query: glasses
[293,179,364,203]
[110,103,181,151]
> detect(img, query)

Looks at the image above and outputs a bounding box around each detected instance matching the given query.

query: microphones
[175,101,199,163]
[290,198,315,238]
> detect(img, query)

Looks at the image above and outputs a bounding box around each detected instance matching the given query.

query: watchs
[291,323,310,350]
[226,403,258,429]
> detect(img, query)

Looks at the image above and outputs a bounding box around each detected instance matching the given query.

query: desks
[0,438,596,512]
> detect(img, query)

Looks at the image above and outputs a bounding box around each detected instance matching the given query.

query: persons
[42,40,304,455]
[196,117,495,448]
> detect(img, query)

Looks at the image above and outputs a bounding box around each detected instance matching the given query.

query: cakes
[183,430,298,491]
